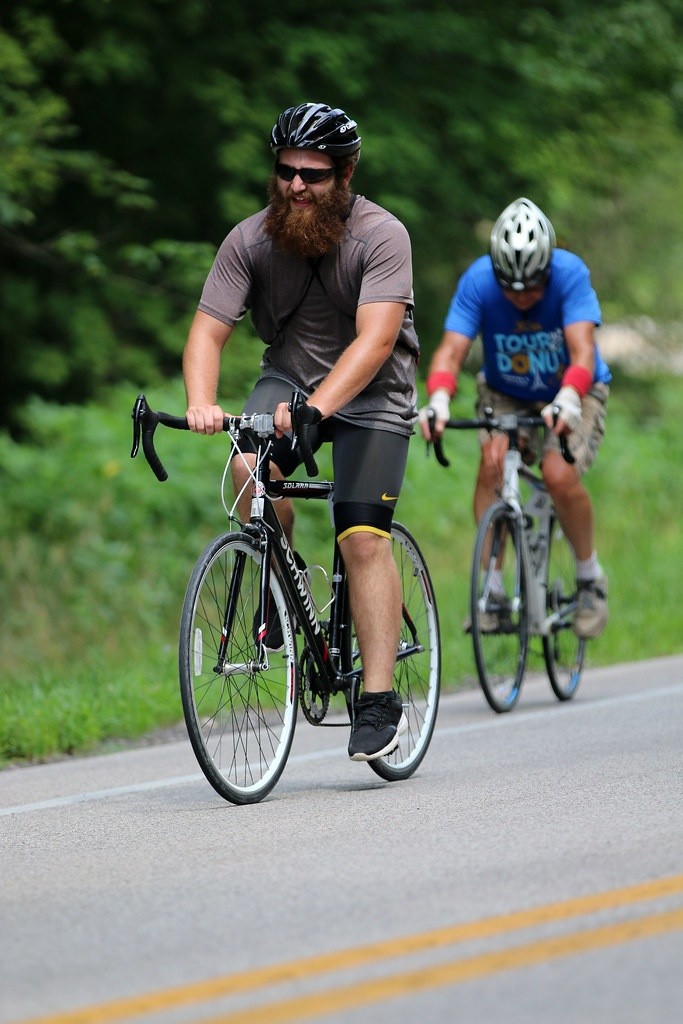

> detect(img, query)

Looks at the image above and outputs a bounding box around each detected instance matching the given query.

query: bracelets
[428,371,458,394]
[564,364,594,394]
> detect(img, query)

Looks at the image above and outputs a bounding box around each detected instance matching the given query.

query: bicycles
[128,386,444,806]
[423,403,590,714]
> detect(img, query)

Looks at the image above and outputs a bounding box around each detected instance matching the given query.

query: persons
[414,197,614,640]
[181,101,420,762]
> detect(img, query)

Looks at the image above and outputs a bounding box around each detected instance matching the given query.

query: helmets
[490,196,556,291]
[269,101,361,169]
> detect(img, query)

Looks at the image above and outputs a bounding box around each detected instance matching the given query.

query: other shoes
[572,570,610,640]
[462,593,513,633]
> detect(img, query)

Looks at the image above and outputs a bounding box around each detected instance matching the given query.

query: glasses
[274,161,335,184]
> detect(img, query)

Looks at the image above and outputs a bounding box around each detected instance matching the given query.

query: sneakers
[252,550,310,653]
[348,688,410,761]
